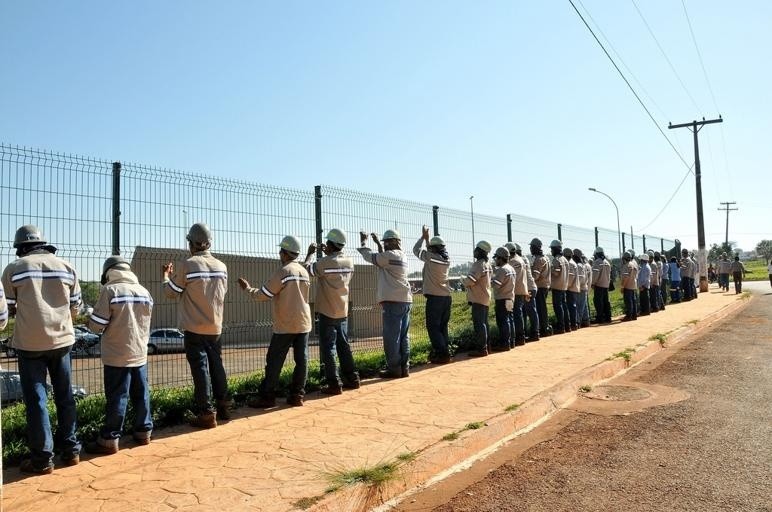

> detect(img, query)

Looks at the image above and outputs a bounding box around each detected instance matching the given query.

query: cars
[0,322,196,360]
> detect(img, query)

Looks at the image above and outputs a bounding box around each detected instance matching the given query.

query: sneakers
[20,459,54,473]
[639,312,649,315]
[581,323,590,327]
[682,298,690,301]
[591,318,606,323]
[554,327,564,333]
[510,342,515,347]
[517,340,525,345]
[190,412,217,428]
[739,291,742,293]
[431,357,450,363]
[342,381,360,388]
[736,293,738,294]
[632,315,637,320]
[287,396,303,405]
[85,434,119,454]
[671,299,680,303]
[726,287,728,291]
[621,316,632,321]
[493,345,510,351]
[660,305,664,309]
[402,369,410,377]
[320,384,342,395]
[218,409,231,420]
[651,307,658,312]
[571,324,578,331]
[566,326,571,332]
[249,396,274,408]
[525,337,539,342]
[723,286,725,290]
[379,370,402,378]
[133,431,151,444]
[61,455,79,465]
[540,325,553,337]
[469,351,487,356]
[476,346,491,354]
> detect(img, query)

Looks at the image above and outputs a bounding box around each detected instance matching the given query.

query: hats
[722,252,727,256]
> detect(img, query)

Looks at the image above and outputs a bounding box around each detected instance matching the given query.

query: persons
[461,240,744,357]
[767,260,772,289]
[355,229,413,379]
[0,280,8,332]
[303,228,360,395]
[84,255,154,455]
[161,223,234,428]
[2,223,82,474]
[412,225,453,363]
[237,235,313,409]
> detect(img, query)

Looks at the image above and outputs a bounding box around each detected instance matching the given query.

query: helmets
[647,251,654,257]
[429,237,446,247]
[477,241,491,253]
[528,238,542,245]
[515,243,521,250]
[638,254,649,260]
[670,256,676,258]
[505,242,516,253]
[573,249,584,256]
[549,240,562,247]
[593,247,603,256]
[682,249,688,254]
[186,223,213,249]
[324,229,345,245]
[654,252,660,256]
[496,247,510,256]
[276,236,301,253]
[628,249,635,255]
[562,248,573,256]
[623,251,631,258]
[13,225,46,249]
[661,255,666,259]
[100,256,132,285]
[380,230,400,241]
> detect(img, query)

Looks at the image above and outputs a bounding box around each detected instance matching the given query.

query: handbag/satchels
[608,280,614,290]
